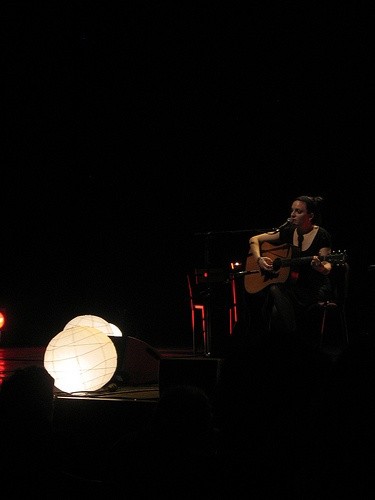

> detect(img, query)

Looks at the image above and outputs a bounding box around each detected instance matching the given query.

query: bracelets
[257,257,261,263]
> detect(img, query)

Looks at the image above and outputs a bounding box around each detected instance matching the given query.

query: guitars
[244,241,348,293]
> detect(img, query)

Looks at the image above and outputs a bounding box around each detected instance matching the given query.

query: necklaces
[296,226,314,235]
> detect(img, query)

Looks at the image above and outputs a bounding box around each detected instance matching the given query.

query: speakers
[109,336,159,386]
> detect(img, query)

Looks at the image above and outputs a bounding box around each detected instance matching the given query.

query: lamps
[44,315,123,395]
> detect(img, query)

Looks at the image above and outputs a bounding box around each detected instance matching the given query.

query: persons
[0,353,375,500]
[248,196,332,339]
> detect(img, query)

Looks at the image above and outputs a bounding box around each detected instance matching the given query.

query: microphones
[275,217,293,233]
